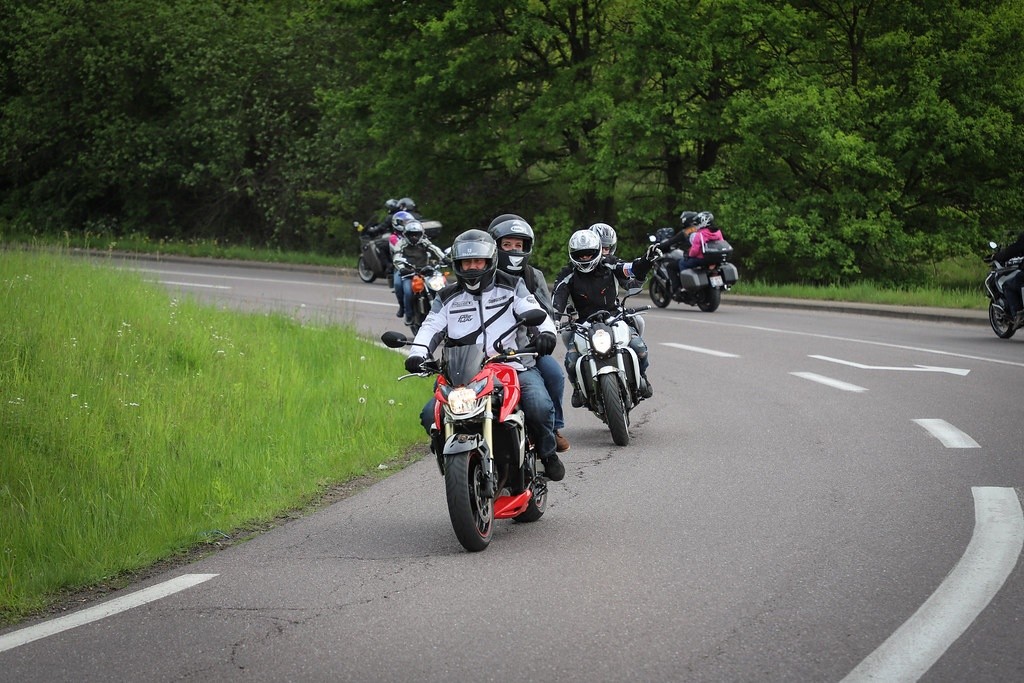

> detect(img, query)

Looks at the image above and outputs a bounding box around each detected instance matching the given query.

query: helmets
[450,229,499,296]
[385,198,400,215]
[395,197,416,212]
[588,223,617,257]
[487,213,535,274]
[402,219,425,247]
[679,211,698,228]
[392,210,416,232]
[692,211,714,230]
[568,229,604,274]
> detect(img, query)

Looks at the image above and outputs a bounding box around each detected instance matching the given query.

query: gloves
[441,255,452,268]
[983,253,995,263]
[530,331,557,357]
[366,226,379,236]
[646,242,664,263]
[399,268,415,279]
[404,356,425,373]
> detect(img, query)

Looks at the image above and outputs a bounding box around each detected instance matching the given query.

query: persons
[984,231,1024,331]
[550,229,655,408]
[554,222,664,351]
[405,229,565,482]
[388,210,452,326]
[419,214,570,453]
[656,210,724,301]
[364,197,423,270]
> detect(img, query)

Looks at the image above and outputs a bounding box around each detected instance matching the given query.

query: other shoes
[553,430,570,453]
[397,308,404,318]
[570,389,584,408]
[404,317,415,326]
[541,452,565,481]
[639,373,654,399]
[1011,315,1021,331]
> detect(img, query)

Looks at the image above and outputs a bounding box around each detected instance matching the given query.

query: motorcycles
[382,308,549,554]
[394,245,453,338]
[648,228,739,312]
[353,220,396,283]
[984,241,1024,340]
[550,288,652,446]
